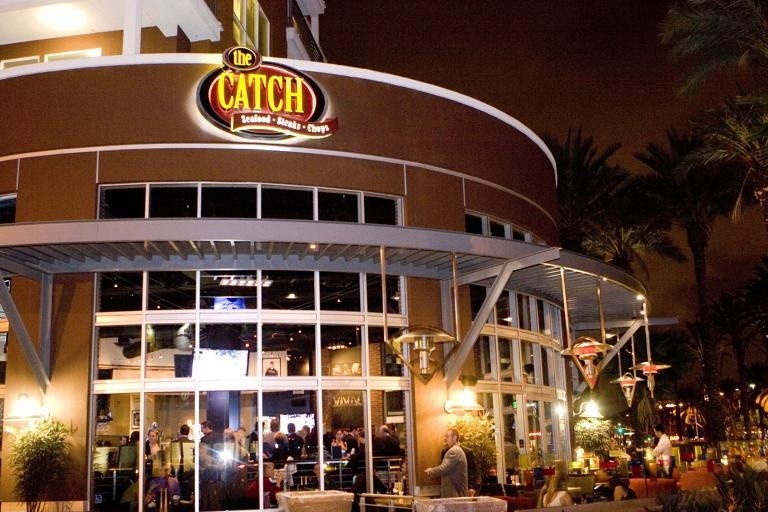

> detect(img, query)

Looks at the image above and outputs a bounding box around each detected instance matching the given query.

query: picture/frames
[262,357,281,377]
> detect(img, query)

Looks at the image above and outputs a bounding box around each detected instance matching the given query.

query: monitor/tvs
[255,415,278,435]
[211,297,245,312]
[191,347,250,377]
[280,411,315,437]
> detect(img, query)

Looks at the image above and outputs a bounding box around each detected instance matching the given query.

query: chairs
[92,442,768,512]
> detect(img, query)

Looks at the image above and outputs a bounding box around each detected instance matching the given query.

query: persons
[264,361,278,377]
[424,430,470,499]
[542,470,574,508]
[89,419,411,512]
[650,423,672,477]
[440,434,478,498]
[599,470,638,502]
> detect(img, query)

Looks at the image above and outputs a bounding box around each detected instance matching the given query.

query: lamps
[610,327,646,409]
[628,303,673,399]
[558,266,615,390]
[573,400,605,419]
[444,389,485,414]
[379,244,464,384]
